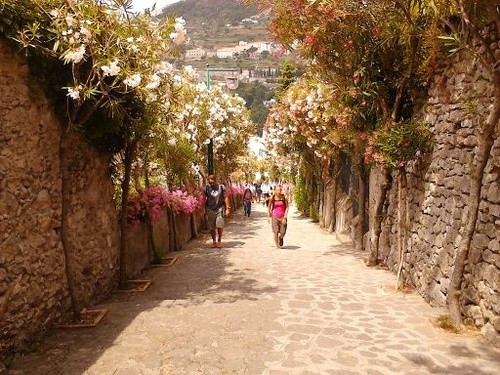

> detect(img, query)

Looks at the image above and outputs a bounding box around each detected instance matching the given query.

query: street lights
[204,64,240,180]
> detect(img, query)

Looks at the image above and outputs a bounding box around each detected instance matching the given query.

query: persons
[242,182,274,217]
[201,174,231,247]
[268,186,288,248]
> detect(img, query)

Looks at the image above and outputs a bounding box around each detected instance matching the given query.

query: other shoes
[217,242,222,247]
[278,236,284,246]
[276,244,281,248]
[245,214,250,218]
[212,243,216,248]
